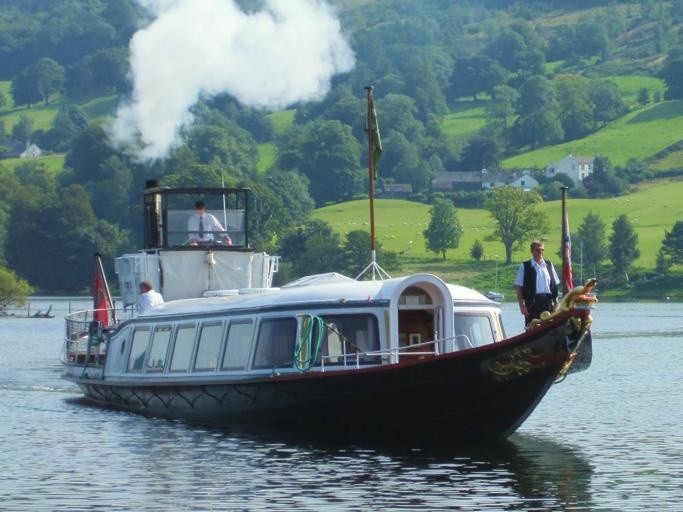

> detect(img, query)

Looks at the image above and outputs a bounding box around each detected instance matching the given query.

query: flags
[91,258,109,327]
[369,98,383,182]
[564,207,574,295]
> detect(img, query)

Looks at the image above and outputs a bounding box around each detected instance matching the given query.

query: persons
[133,281,167,314]
[515,241,561,332]
[187,200,232,246]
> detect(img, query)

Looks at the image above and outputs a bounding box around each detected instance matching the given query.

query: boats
[60,83,600,452]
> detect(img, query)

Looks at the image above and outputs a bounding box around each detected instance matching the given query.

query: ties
[198,216,204,238]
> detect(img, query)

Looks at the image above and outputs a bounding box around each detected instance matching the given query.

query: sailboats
[555,241,598,300]
[484,255,507,303]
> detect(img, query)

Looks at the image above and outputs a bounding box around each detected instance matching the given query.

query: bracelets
[554,302,559,304]
